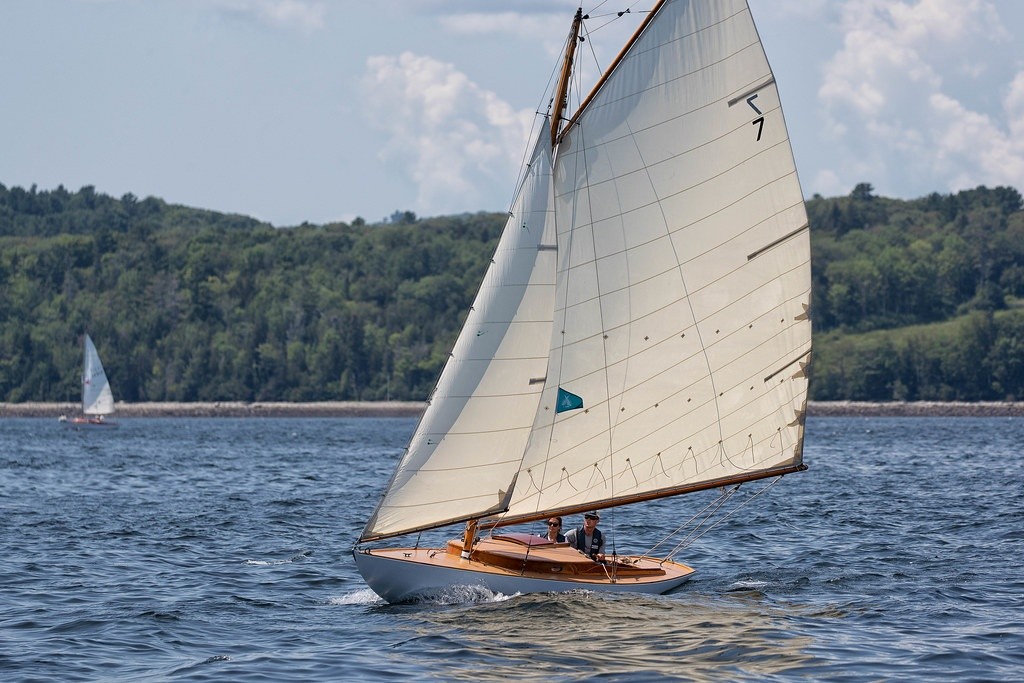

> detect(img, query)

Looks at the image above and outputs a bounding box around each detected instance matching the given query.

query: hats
[583,511,600,519]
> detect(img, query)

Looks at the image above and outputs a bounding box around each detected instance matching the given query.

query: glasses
[547,522,558,527]
[584,515,596,520]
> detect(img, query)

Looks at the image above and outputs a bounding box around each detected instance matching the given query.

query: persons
[565,510,606,564]
[96,414,104,421]
[537,516,565,543]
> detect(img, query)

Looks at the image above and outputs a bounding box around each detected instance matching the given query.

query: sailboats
[351,0,813,606]
[60,333,120,430]
